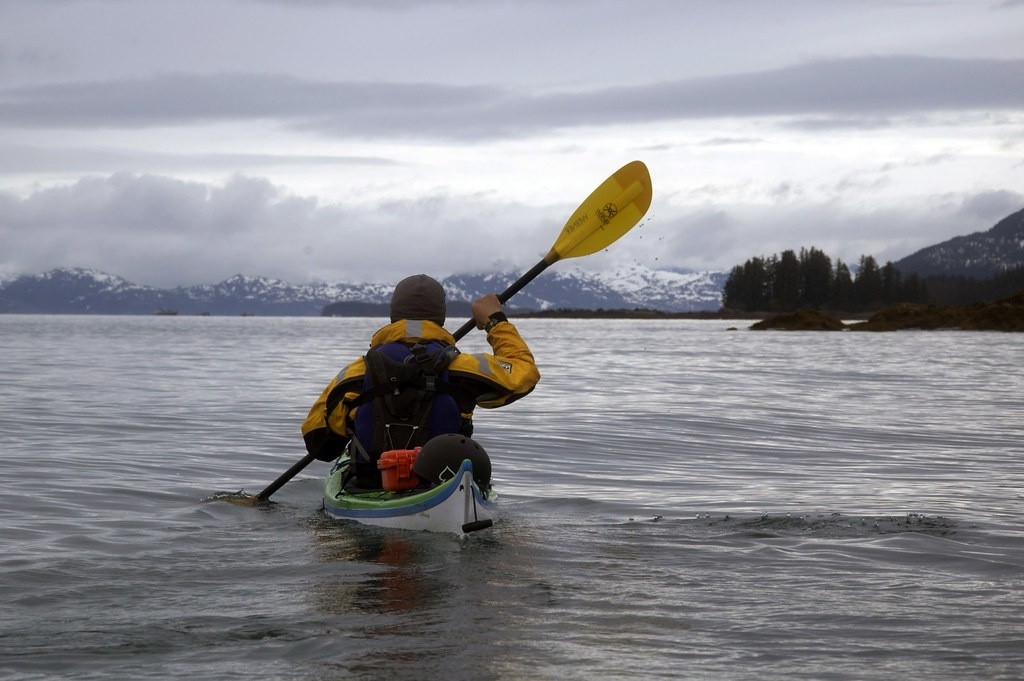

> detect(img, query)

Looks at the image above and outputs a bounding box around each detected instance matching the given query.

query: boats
[320,423,500,544]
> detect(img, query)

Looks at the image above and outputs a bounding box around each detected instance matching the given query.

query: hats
[390,274,446,326]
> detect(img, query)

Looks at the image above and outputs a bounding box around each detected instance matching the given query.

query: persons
[303,274,541,492]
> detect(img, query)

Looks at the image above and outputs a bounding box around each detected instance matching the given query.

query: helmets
[414,434,491,492]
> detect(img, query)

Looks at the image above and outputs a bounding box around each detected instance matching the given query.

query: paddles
[235,159,653,503]
